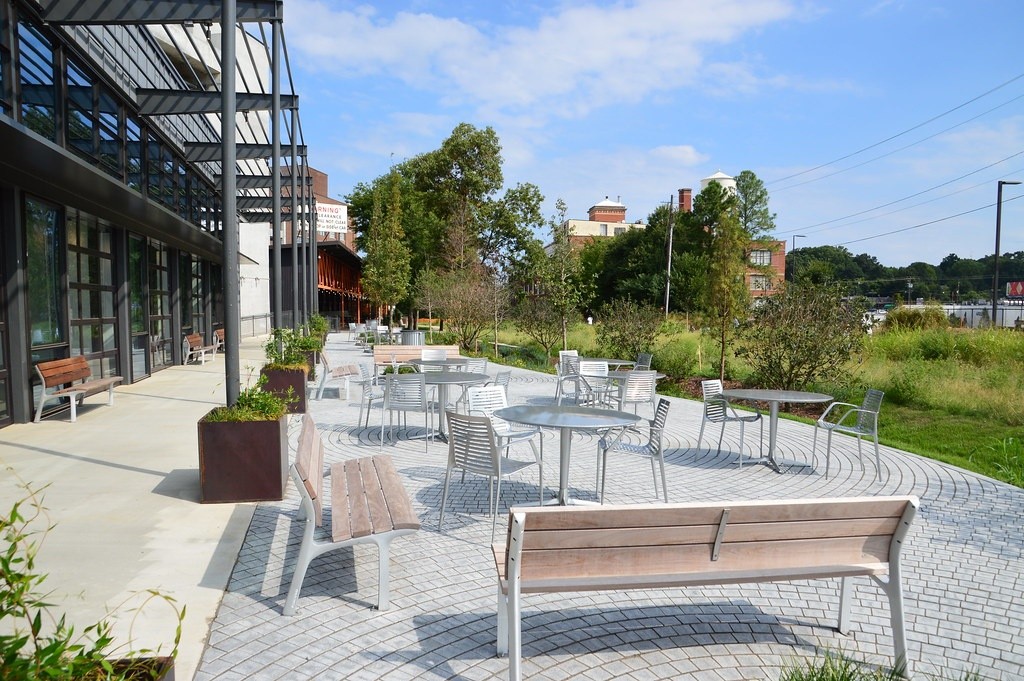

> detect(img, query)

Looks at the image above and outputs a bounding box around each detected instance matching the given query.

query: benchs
[183,332,220,366]
[492,488,920,681]
[316,350,358,403]
[212,328,225,360]
[33,355,123,422]
[281,412,422,617]
[373,346,424,386]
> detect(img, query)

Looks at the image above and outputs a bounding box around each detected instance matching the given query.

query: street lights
[992,181,1023,326]
[791,234,806,298]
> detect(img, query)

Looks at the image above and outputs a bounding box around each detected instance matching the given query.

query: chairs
[695,379,764,471]
[437,386,546,533]
[357,358,511,452]
[348,323,366,340]
[810,388,885,482]
[595,398,671,506]
[553,349,658,420]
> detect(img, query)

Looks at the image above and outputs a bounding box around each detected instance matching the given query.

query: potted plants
[196,364,301,503]
[259,306,334,414]
[0,461,189,681]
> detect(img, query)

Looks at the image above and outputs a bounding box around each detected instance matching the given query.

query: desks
[380,333,402,344]
[577,358,666,411]
[354,330,376,346]
[493,403,642,508]
[722,389,834,474]
[398,357,491,445]
